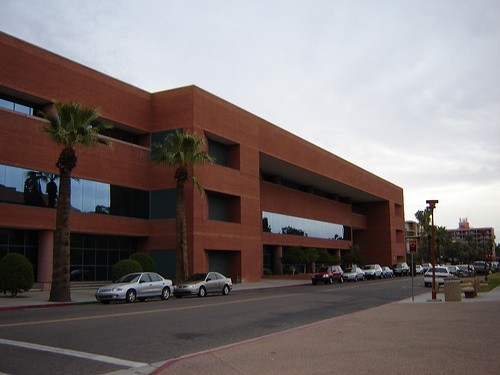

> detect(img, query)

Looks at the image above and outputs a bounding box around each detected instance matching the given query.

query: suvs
[311,264,345,285]
[362,264,384,280]
[390,262,411,277]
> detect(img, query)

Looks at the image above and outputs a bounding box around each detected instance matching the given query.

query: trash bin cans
[443,276,461,301]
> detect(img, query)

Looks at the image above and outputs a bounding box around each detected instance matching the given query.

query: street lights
[425,199,439,300]
[482,228,488,281]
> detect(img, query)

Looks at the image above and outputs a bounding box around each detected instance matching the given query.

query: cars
[95,271,172,304]
[423,266,454,287]
[415,261,500,278]
[382,266,393,279]
[343,267,365,283]
[172,271,234,298]
